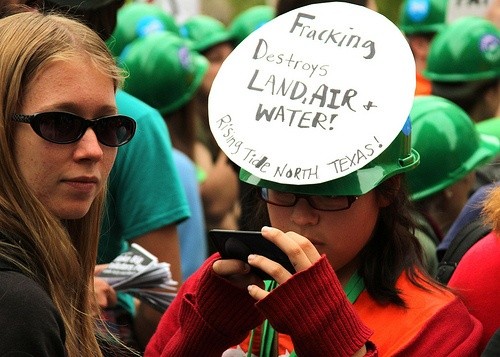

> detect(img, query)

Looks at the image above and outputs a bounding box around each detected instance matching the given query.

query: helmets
[474,118,500,140]
[180,15,236,52]
[104,2,179,56]
[422,17,500,82]
[399,0,447,34]
[405,95,500,201]
[228,5,278,46]
[239,115,420,195]
[117,31,209,112]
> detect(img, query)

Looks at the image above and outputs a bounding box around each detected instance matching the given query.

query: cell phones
[209,229,296,280]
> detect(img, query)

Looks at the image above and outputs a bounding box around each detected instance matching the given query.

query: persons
[0,10,136,357]
[102,0,500,357]
[0,0,192,357]
[143,0,483,357]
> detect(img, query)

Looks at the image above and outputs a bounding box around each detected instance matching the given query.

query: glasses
[258,187,376,211]
[13,111,136,147]
